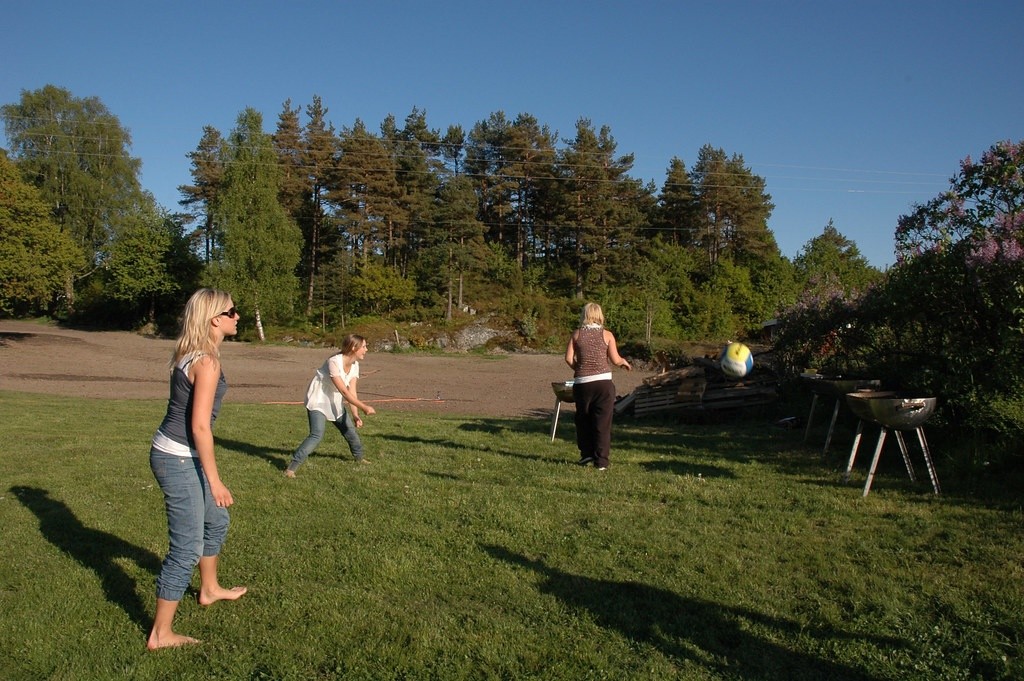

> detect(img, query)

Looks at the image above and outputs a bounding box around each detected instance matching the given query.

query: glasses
[220,307,236,318]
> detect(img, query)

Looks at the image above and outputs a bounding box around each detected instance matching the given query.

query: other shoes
[578,456,593,464]
[596,465,607,471]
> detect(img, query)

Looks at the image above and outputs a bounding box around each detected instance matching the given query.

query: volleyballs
[720,342,753,377]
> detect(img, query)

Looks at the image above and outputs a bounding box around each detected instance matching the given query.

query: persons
[148,287,247,649]
[283,334,377,478]
[565,301,632,471]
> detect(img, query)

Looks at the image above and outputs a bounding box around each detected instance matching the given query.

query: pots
[845,388,937,431]
[816,376,883,398]
